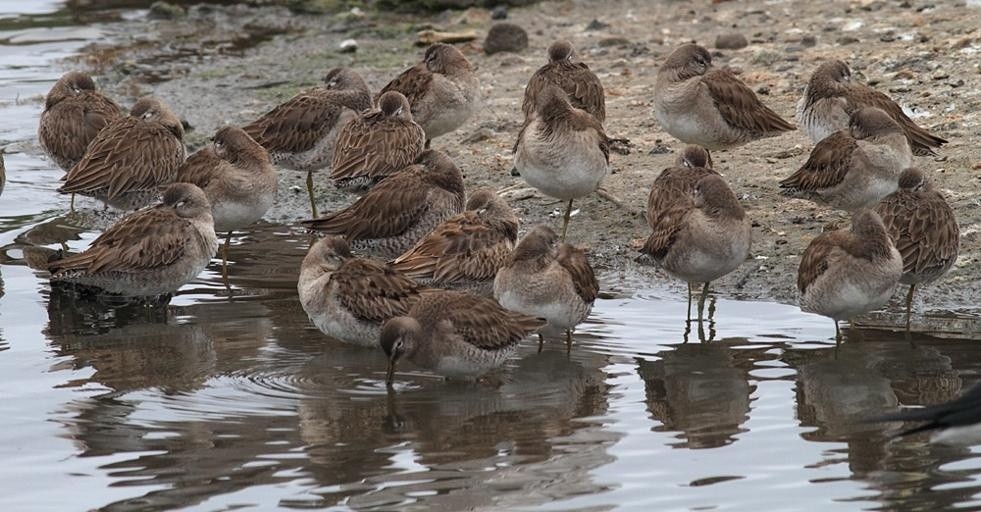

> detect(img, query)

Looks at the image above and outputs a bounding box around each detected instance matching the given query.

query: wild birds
[636,324,961,464]
[44,42,626,348]
[781,61,960,346]
[378,291,550,386]
[641,41,798,329]
